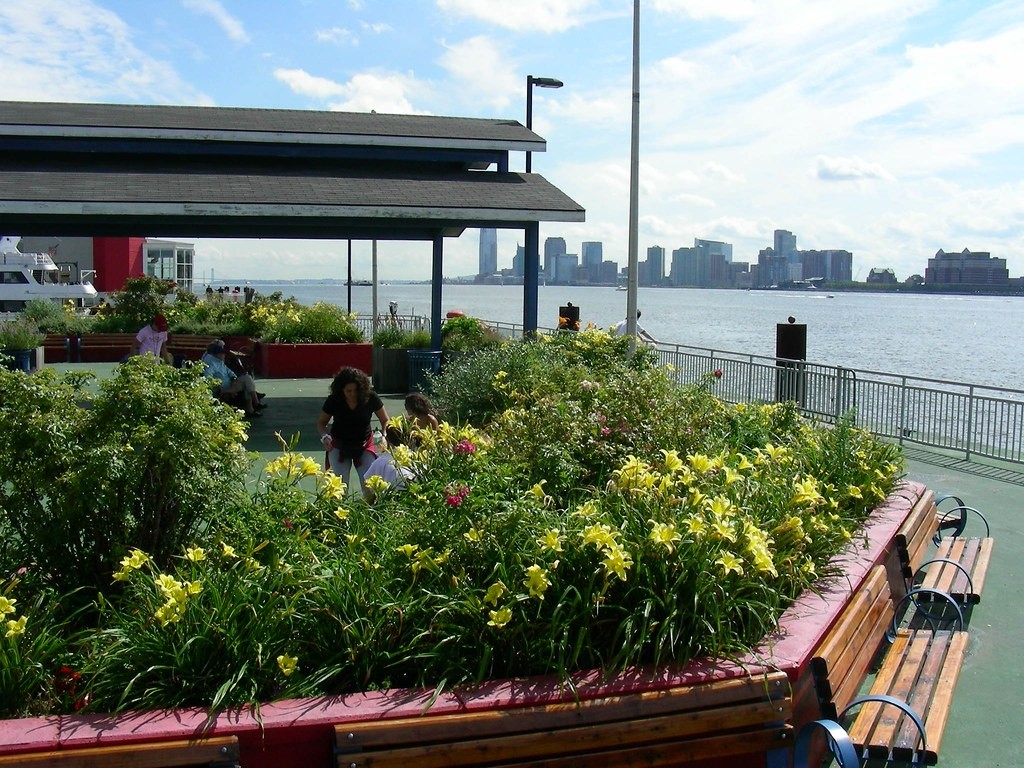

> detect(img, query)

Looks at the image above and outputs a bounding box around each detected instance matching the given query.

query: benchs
[932,495,967,543]
[327,671,860,768]
[77,332,140,362]
[0,735,242,768]
[809,565,969,768]
[41,334,73,363]
[161,334,224,364]
[895,489,994,633]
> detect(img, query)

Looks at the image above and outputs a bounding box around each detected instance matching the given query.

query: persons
[612,309,659,345]
[62,296,105,317]
[130,315,269,419]
[317,368,440,505]
[205,284,239,303]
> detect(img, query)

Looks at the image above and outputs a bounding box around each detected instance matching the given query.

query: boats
[0,235,99,324]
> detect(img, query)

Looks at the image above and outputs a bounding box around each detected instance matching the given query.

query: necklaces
[153,329,159,356]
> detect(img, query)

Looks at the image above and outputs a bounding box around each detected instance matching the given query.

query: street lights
[524,73,564,172]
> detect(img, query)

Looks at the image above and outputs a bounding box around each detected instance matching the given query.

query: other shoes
[245,410,263,418]
[257,393,265,400]
[257,403,267,410]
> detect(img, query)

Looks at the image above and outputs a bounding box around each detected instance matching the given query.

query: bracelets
[320,433,333,444]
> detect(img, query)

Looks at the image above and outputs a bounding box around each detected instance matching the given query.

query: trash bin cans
[0,348,32,372]
[406,349,443,397]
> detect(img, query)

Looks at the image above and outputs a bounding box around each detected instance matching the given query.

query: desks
[89,306,116,315]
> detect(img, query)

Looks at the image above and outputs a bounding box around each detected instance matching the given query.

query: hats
[213,347,228,353]
[155,316,167,331]
